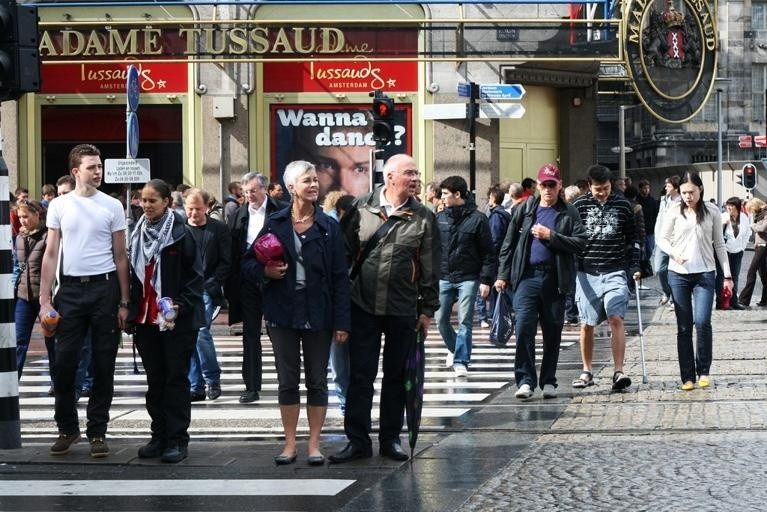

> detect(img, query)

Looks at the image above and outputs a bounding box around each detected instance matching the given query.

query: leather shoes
[239,390,260,402]
[380,442,409,460]
[209,384,220,400]
[190,389,206,401]
[275,448,297,465]
[328,442,373,462]
[308,457,325,465]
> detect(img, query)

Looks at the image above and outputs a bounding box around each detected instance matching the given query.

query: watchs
[119,301,131,310]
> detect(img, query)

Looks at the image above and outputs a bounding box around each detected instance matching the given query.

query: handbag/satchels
[254,232,283,264]
[489,290,514,346]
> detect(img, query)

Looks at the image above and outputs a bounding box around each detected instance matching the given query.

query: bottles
[722,283,731,309]
[41,310,60,337]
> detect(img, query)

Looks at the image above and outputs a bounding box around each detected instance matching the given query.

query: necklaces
[291,204,314,223]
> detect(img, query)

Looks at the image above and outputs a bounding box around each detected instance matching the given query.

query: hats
[537,164,561,184]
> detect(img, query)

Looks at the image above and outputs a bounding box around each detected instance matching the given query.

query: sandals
[572,371,594,387]
[612,370,631,390]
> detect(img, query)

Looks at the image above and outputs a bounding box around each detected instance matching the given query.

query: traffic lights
[371,97,396,142]
[744,165,756,188]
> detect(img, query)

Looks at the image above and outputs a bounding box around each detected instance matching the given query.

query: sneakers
[50,434,82,454]
[455,365,467,377]
[446,352,455,367]
[682,385,693,390]
[543,384,556,398]
[138,437,163,459]
[89,437,110,457]
[161,442,187,462]
[516,384,534,398]
[699,378,710,387]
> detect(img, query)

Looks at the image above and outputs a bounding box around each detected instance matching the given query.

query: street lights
[712,75,731,210]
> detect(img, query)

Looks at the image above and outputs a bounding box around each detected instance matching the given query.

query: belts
[64,274,106,284]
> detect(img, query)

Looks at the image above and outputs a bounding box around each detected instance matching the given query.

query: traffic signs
[754,135,767,141]
[479,103,527,118]
[755,142,767,148]
[738,135,752,142]
[739,142,753,148]
[420,100,470,121]
[103,156,151,184]
[457,82,471,97]
[479,83,527,99]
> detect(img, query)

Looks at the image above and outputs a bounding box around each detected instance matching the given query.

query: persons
[284,109,374,202]
[9,144,441,464]
[634,171,767,390]
[433,163,646,399]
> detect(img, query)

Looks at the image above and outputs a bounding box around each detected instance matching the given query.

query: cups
[156,296,176,321]
